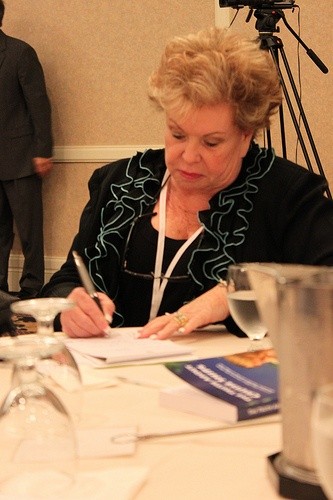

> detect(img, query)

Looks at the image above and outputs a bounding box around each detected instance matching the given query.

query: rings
[176,316,189,326]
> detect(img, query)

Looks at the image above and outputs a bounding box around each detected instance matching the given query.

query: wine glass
[10,298,84,427]
[0,335,84,499]
[226,266,268,351]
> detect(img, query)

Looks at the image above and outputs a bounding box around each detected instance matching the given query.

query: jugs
[241,262,333,486]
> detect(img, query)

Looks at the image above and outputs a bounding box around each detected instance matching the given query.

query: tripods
[253,8,332,202]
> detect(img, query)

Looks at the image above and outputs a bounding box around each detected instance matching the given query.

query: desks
[1,324,286,500]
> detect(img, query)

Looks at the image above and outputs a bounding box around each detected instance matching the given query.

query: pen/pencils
[72,251,104,315]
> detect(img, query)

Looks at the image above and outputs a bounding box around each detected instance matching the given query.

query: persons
[0,0,54,300]
[37,26,333,340]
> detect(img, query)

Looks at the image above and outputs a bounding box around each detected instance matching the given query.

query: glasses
[123,213,205,279]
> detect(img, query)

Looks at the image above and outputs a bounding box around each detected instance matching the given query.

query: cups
[309,382,333,500]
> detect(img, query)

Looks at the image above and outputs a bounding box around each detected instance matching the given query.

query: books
[160,349,284,423]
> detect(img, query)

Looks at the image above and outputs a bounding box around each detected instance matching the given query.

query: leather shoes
[17,287,40,300]
